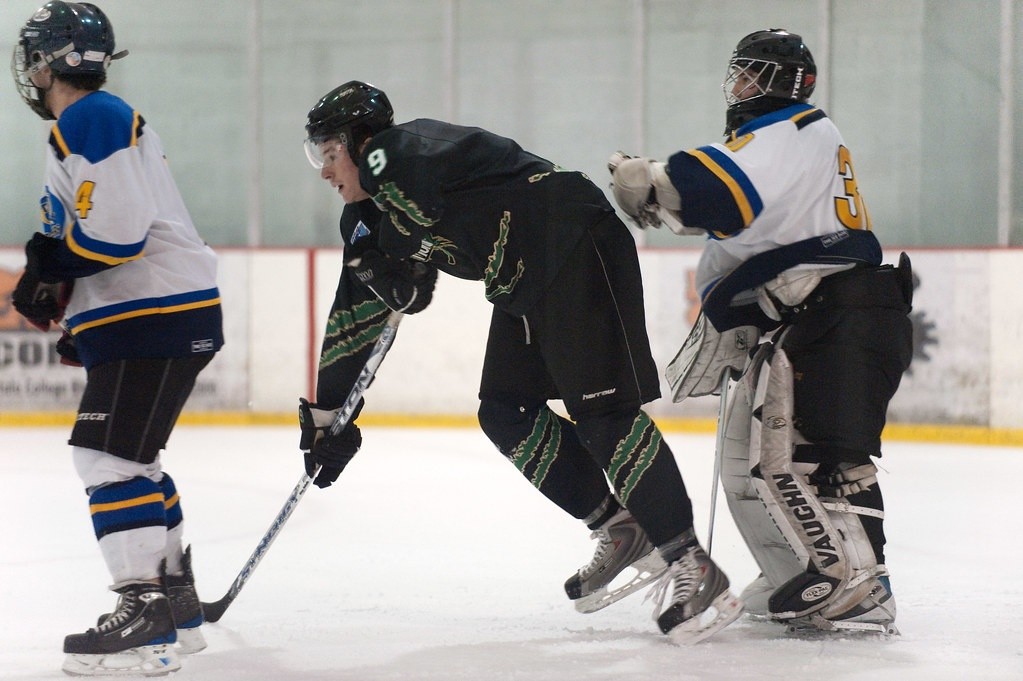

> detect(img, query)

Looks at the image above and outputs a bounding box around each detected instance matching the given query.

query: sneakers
[163,545,203,631]
[565,505,655,601]
[658,534,728,634]
[66,556,177,653]
[790,564,890,627]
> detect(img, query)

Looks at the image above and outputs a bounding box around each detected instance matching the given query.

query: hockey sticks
[200,235,437,625]
[38,290,73,336]
[706,365,729,557]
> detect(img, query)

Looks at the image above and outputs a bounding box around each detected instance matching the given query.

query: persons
[612,27,913,631]
[298,80,743,645]
[10,1,223,677]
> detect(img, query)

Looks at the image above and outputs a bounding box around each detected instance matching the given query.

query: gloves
[11,231,112,331]
[299,398,362,489]
[392,275,431,313]
[612,158,682,230]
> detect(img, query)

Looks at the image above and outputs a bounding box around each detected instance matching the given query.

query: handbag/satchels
[769,556,849,619]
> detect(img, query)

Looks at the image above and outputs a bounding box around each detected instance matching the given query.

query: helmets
[10,0,127,120]
[721,28,817,136]
[304,81,394,169]
